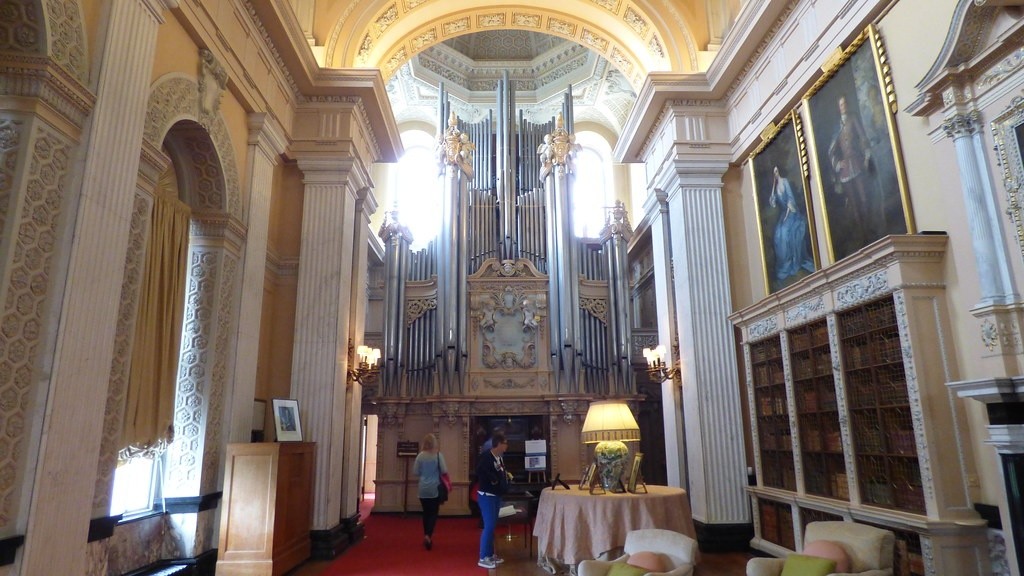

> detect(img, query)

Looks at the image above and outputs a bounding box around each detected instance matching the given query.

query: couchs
[745,521,895,576]
[577,527,697,576]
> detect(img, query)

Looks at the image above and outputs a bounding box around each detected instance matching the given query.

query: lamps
[581,399,641,491]
[642,343,682,385]
[346,339,381,390]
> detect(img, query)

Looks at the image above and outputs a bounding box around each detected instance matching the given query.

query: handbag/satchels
[436,451,452,504]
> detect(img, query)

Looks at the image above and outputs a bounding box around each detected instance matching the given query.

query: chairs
[470,481,536,559]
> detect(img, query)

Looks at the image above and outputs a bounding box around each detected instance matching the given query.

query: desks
[535,484,698,576]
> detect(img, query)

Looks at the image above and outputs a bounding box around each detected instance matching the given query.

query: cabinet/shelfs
[730,235,991,576]
[212,441,318,576]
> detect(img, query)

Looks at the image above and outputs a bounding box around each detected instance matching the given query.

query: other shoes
[489,554,504,564]
[423,536,432,550]
[477,557,496,569]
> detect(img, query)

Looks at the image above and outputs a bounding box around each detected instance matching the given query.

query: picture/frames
[797,21,919,266]
[627,452,649,495]
[551,472,569,489]
[271,397,304,442]
[990,91,1024,261]
[747,109,822,297]
[590,463,605,495]
[578,464,590,491]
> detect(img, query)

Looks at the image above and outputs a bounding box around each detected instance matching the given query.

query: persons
[282,409,295,431]
[413,434,452,550]
[470,427,509,570]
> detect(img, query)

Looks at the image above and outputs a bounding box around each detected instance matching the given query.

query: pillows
[605,562,647,576]
[780,553,836,576]
[802,540,849,573]
[626,551,665,573]
